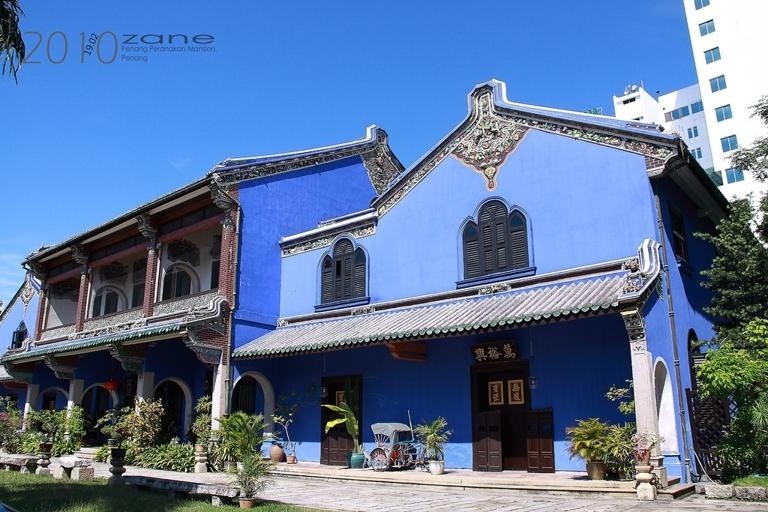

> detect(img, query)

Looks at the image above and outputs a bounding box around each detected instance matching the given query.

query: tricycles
[369,421,445,471]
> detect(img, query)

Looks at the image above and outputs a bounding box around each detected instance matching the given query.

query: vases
[269,443,284,462]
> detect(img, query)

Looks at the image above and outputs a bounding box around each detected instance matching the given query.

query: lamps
[527,374,537,391]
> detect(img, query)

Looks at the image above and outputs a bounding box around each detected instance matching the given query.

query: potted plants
[558,375,639,483]
[108,413,147,462]
[406,413,455,477]
[204,412,268,510]
[269,412,296,464]
[320,402,365,469]
[36,418,62,452]
